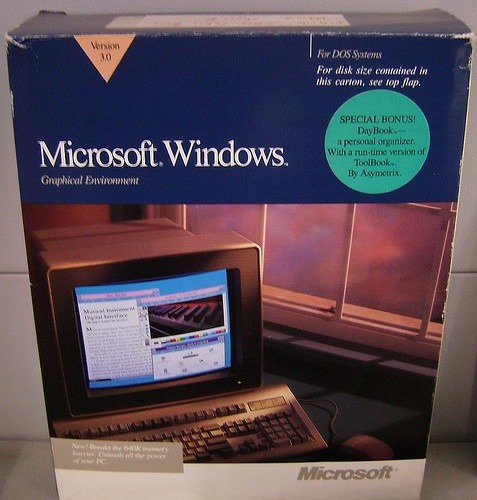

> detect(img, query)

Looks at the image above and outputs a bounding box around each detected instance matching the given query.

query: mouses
[338,434,395,461]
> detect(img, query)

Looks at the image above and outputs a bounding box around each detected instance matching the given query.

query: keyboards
[49,383,329,465]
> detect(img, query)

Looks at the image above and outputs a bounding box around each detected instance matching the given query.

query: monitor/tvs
[32,230,264,420]
